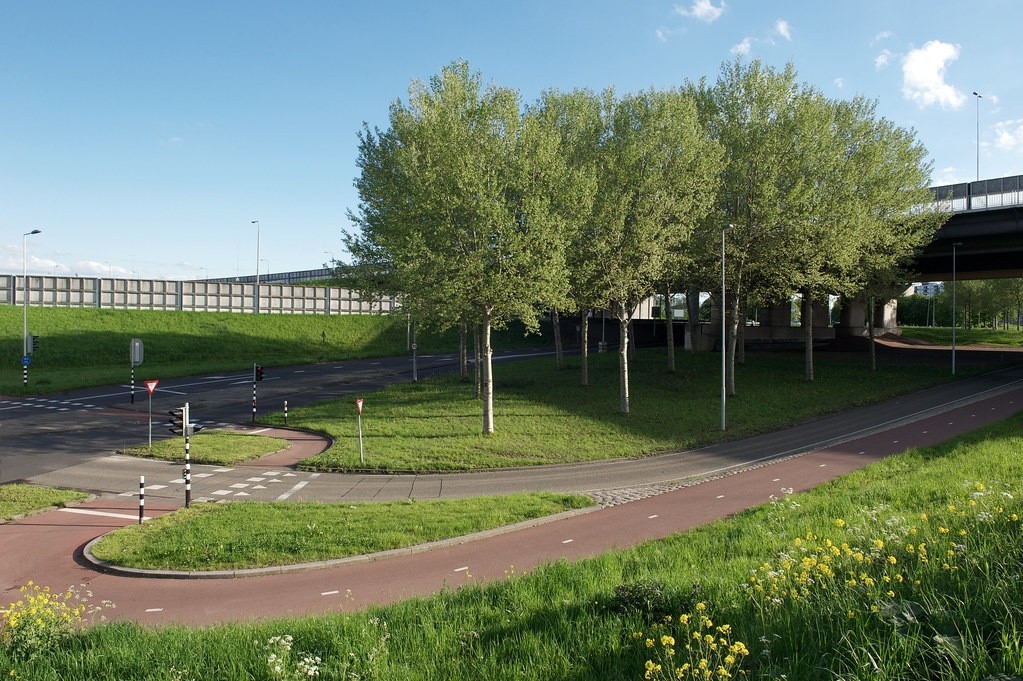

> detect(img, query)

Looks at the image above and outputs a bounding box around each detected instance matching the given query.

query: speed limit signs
[411,342,417,350]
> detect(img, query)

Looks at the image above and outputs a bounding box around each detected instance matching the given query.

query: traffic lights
[189,423,205,435]
[168,406,185,437]
[255,365,264,381]
[27,335,40,356]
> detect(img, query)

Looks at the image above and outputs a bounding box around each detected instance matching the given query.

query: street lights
[251,220,261,313]
[721,223,735,431]
[200,267,208,279]
[972,91,984,182]
[324,250,336,274]
[260,258,269,281]
[952,241,964,376]
[22,228,42,389]
